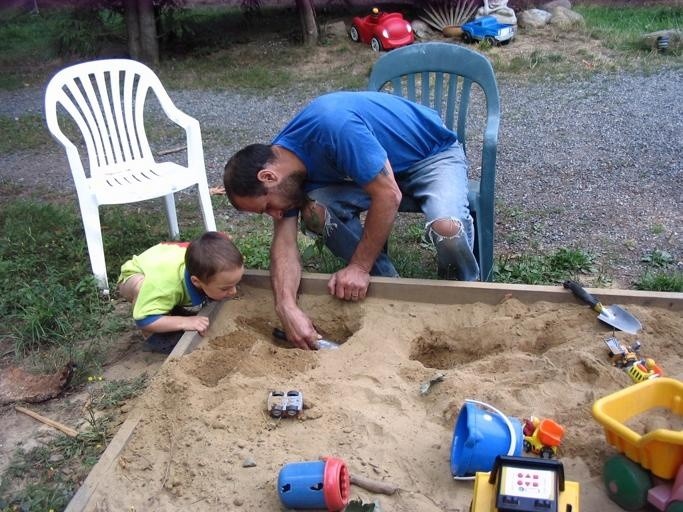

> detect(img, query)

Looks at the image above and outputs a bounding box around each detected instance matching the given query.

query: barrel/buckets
[450,399,525,482]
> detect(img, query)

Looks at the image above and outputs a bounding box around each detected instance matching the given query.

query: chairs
[45,57,217,299]
[366,43,502,283]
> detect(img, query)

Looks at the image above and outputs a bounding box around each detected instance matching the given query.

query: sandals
[143,333,172,353]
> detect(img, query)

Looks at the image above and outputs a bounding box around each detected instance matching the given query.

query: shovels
[563,279,642,334]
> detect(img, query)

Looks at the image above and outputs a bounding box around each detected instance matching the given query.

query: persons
[223,90,480,351]
[117,231,245,355]
